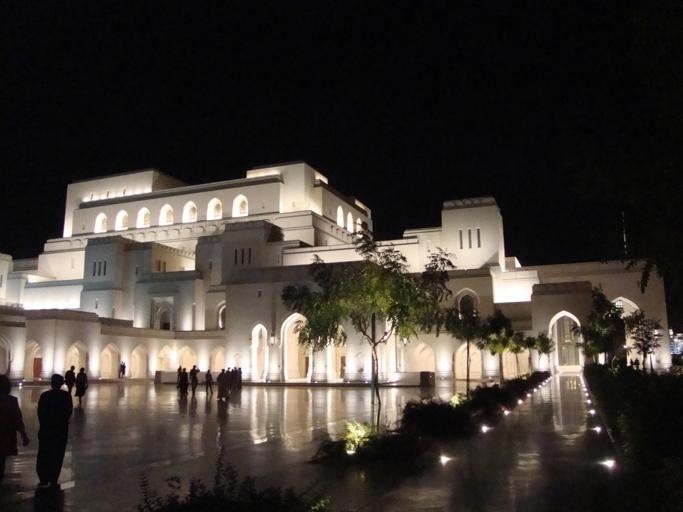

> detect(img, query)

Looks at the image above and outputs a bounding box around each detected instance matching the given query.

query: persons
[36,374,73,488]
[65,366,76,392]
[119,361,126,379]
[0,374,30,482]
[176,365,242,402]
[75,368,89,404]
